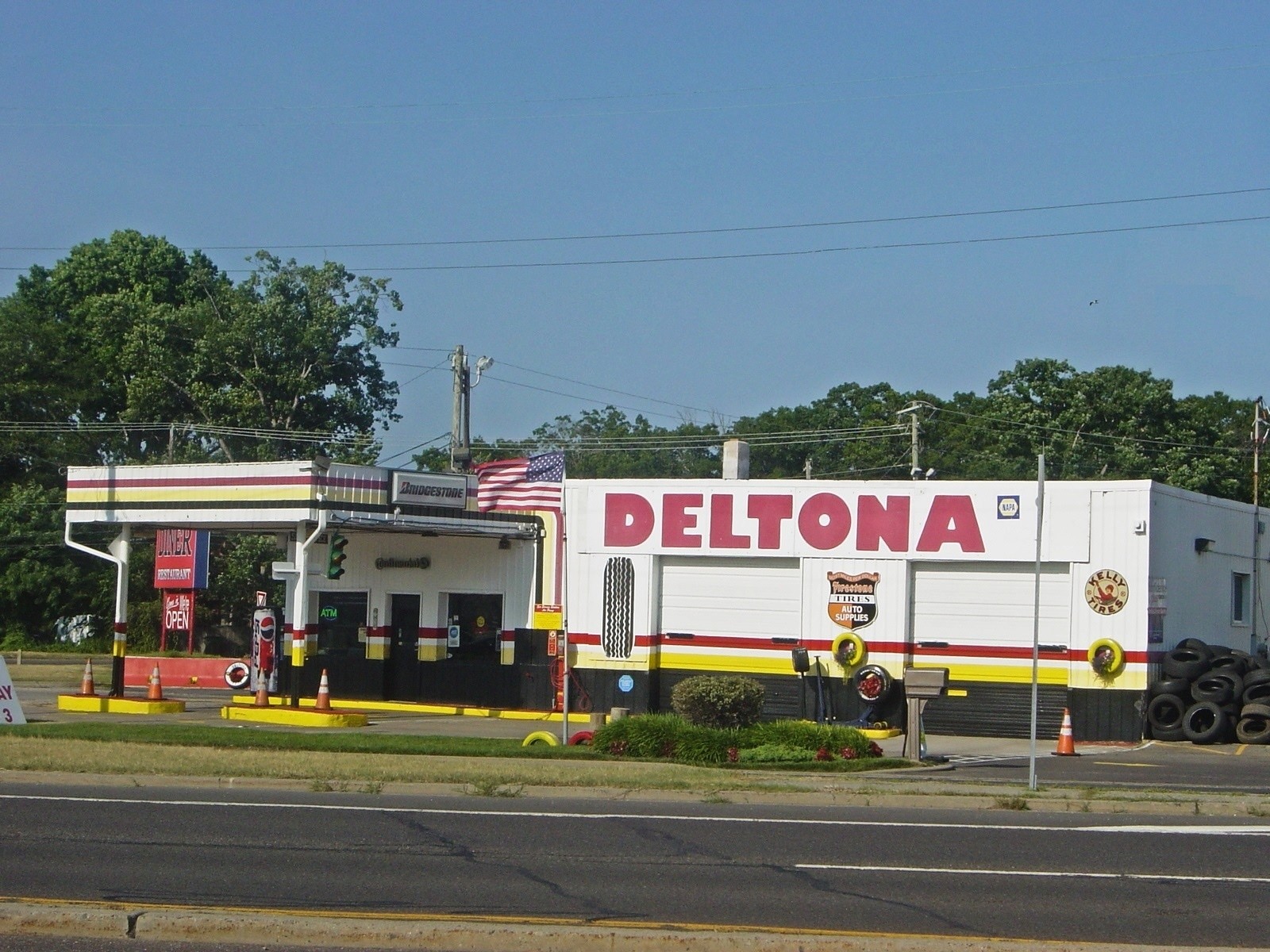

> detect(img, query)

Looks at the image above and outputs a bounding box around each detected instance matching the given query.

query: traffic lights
[328,532,349,582]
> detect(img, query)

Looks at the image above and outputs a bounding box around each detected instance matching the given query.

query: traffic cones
[75,657,101,696]
[312,668,335,711]
[143,661,169,700]
[1051,708,1085,757]
[249,667,276,708]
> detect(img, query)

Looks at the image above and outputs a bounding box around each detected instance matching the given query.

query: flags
[469,451,566,515]
